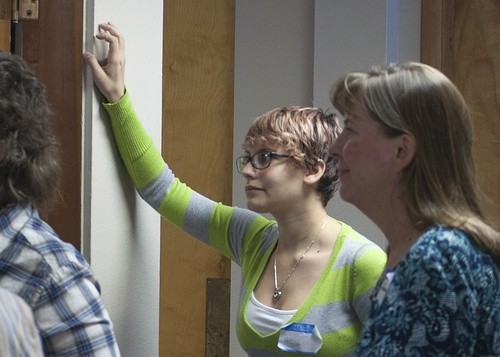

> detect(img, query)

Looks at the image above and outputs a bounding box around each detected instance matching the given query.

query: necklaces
[273,219,330,302]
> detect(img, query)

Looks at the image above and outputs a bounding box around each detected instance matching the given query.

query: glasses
[236,150,293,174]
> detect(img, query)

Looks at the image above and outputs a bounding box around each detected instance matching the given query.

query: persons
[0,50,122,357]
[84,21,387,357]
[328,61,500,357]
